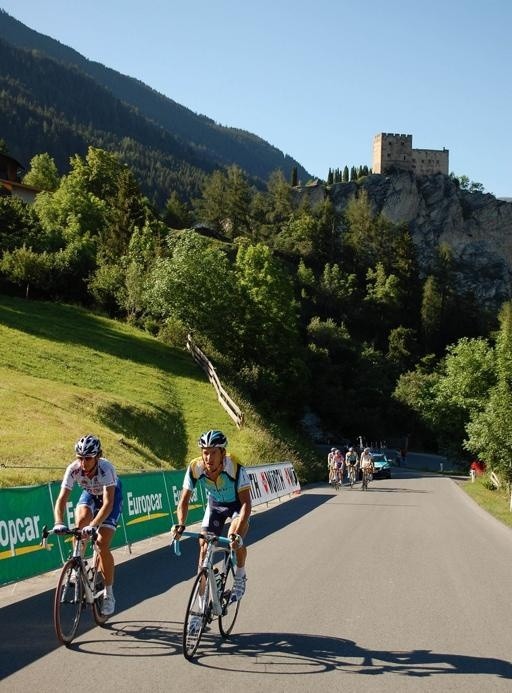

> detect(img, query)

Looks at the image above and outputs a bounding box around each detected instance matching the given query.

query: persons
[332,449,344,484]
[327,447,338,484]
[395,447,401,466]
[344,446,359,485]
[399,444,408,465]
[359,447,374,487]
[51,434,123,616]
[170,429,252,633]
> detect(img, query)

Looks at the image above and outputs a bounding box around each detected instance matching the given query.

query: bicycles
[40,524,110,644]
[328,464,372,492]
[171,525,240,661]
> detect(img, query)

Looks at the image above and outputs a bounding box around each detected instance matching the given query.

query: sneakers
[231,575,247,601]
[101,594,115,615]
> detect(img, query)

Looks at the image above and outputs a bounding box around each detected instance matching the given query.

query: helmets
[331,447,371,453]
[198,430,228,447]
[75,435,102,458]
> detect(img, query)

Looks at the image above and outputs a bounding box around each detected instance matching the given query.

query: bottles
[84,560,94,590]
[213,568,222,594]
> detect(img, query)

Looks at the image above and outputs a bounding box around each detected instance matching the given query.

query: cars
[358,454,392,479]
